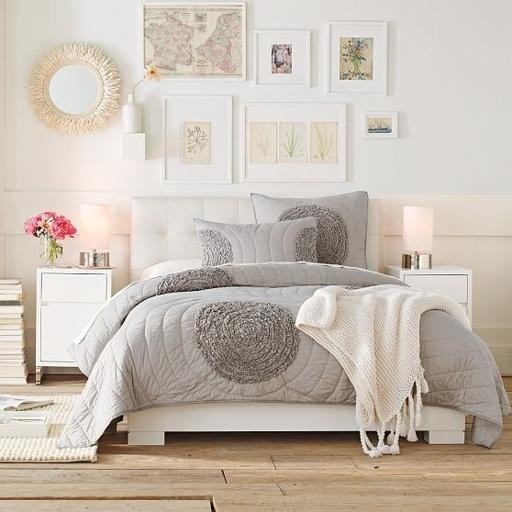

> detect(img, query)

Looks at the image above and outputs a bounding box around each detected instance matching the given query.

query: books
[0,277,31,385]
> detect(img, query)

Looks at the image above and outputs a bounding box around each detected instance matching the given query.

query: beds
[56,191,512,449]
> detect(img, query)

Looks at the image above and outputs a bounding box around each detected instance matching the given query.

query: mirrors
[25,40,121,135]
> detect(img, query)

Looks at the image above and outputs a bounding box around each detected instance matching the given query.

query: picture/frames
[363,111,398,140]
[139,1,246,82]
[326,17,388,98]
[162,94,232,185]
[239,101,346,183]
[254,29,310,88]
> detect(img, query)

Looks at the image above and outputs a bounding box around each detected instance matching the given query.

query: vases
[45,235,50,266]
[122,93,143,133]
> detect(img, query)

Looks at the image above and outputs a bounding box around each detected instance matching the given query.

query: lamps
[402,206,432,269]
[81,203,111,268]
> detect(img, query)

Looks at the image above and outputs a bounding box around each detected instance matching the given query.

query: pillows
[191,216,318,267]
[250,191,369,270]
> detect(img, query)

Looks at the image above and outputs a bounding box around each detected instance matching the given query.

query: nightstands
[35,265,112,385]
[385,265,473,328]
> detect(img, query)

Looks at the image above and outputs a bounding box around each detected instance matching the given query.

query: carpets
[0,393,98,463]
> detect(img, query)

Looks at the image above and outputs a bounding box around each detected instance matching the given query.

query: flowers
[132,65,161,95]
[23,210,77,263]
